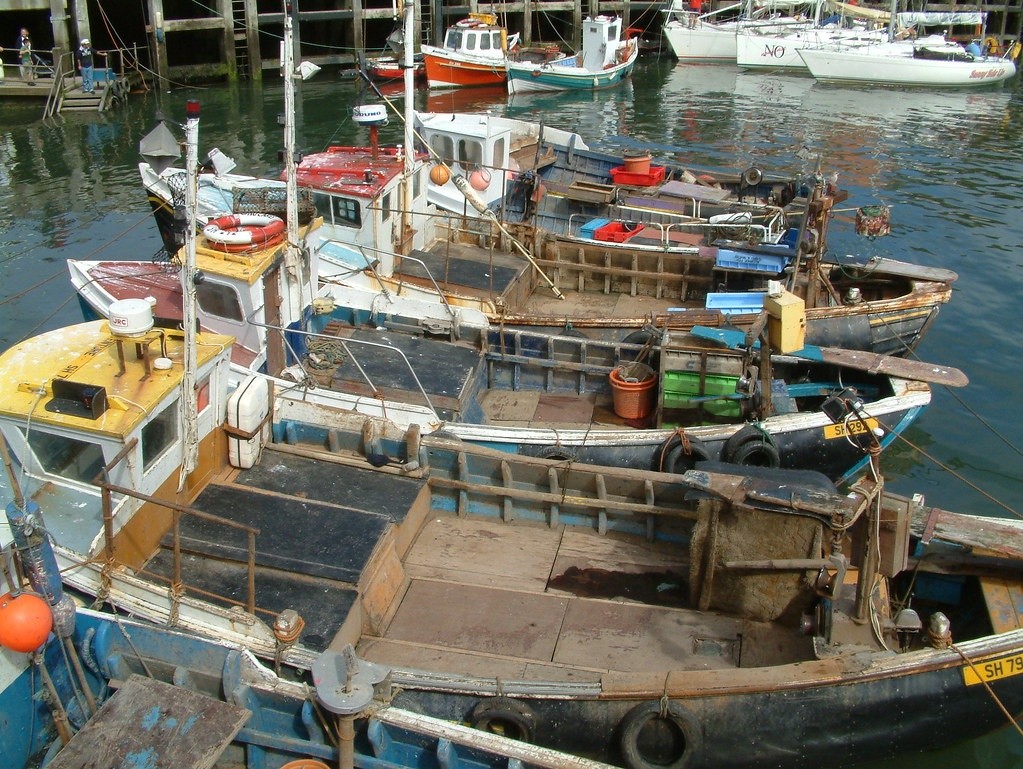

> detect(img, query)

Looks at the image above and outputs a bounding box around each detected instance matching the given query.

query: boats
[136,1,961,357]
[278,109,852,261]
[496,13,642,97]
[0,426,628,769]
[356,50,427,83]
[735,0,965,69]
[657,0,816,60]
[60,15,970,491]
[418,10,567,90]
[1,95,1023,769]
[794,22,1018,88]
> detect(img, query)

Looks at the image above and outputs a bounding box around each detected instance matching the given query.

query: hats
[81,39,89,45]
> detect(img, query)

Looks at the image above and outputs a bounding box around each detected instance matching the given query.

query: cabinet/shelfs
[658,330,751,430]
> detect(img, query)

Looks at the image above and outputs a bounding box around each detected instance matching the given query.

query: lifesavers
[558,330,587,339]
[534,446,581,463]
[1011,43,1022,59]
[456,18,481,28]
[721,425,782,468]
[204,212,286,253]
[469,697,541,766]
[985,37,997,54]
[616,699,703,769]
[655,434,713,473]
[112,80,123,98]
[623,330,661,346]
[109,96,120,108]
[122,78,130,92]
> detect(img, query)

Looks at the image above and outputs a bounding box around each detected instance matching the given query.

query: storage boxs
[579,219,643,244]
[610,166,666,185]
[717,250,789,274]
[707,291,767,314]
[662,355,743,429]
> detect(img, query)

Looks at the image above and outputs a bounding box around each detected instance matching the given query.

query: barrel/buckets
[621,150,653,175]
[609,366,656,418]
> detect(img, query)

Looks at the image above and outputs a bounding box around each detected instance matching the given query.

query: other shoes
[83,91,88,93]
[28,82,36,86]
[90,91,95,94]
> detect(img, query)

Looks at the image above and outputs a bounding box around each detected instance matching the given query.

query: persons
[21,39,37,86]
[77,38,107,94]
[17,29,31,80]
